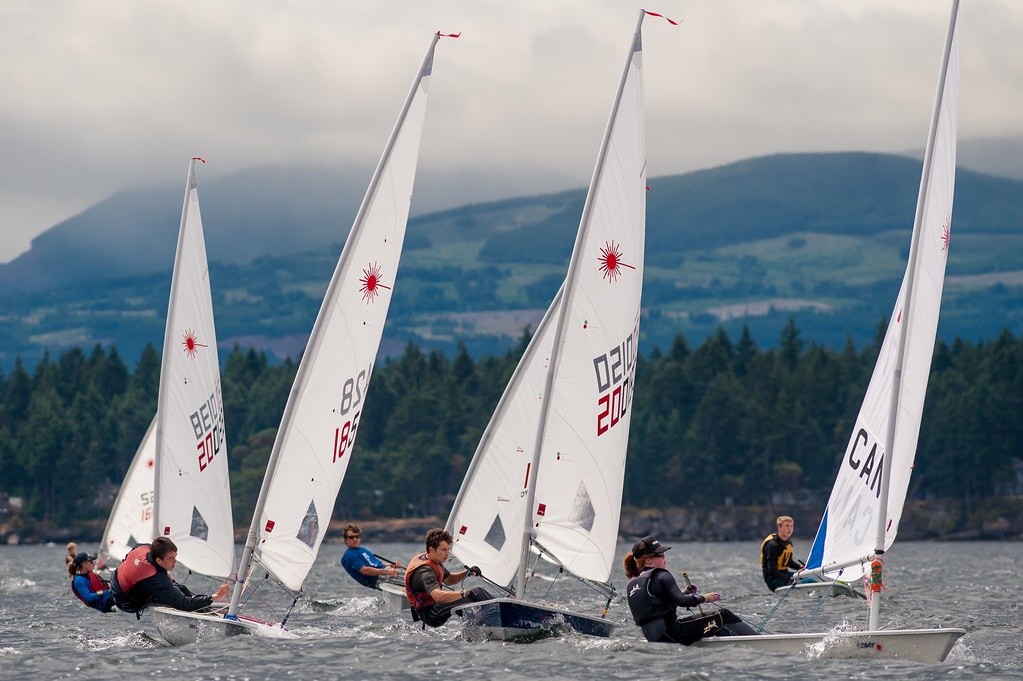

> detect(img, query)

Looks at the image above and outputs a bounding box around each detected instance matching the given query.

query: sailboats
[89,5,685,646]
[686,1,969,662]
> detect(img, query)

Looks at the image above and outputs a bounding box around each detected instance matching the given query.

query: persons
[404,528,494,627]
[760,515,804,594]
[65,543,78,564]
[341,524,406,591]
[66,552,117,614]
[623,536,761,646]
[110,537,230,613]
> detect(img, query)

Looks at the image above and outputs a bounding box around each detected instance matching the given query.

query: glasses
[642,553,665,557]
[348,535,360,539]
[86,561,94,564]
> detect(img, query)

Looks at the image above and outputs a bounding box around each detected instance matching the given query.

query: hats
[632,535,672,559]
[72,551,98,562]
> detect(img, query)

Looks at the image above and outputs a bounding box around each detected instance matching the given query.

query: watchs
[460,590,464,598]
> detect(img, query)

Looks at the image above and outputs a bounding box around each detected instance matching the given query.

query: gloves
[682,584,697,594]
[702,592,721,603]
[467,586,487,601]
[468,565,482,577]
